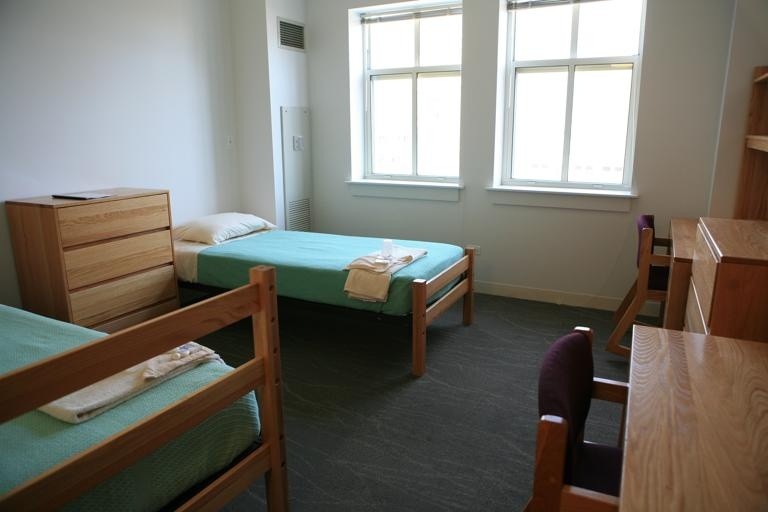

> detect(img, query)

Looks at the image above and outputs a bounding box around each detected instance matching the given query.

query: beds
[173,230,475,377]
[0,265,289,512]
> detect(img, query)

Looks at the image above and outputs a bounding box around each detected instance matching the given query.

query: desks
[619,325,768,512]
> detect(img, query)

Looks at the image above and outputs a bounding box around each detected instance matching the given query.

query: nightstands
[5,188,181,335]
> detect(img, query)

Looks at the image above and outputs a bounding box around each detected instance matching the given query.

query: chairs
[605,214,672,358]
[525,326,628,512]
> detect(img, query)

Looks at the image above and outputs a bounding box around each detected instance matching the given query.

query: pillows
[172,212,277,245]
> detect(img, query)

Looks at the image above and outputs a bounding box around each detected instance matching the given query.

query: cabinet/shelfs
[683,218,768,344]
[662,64,768,332]
